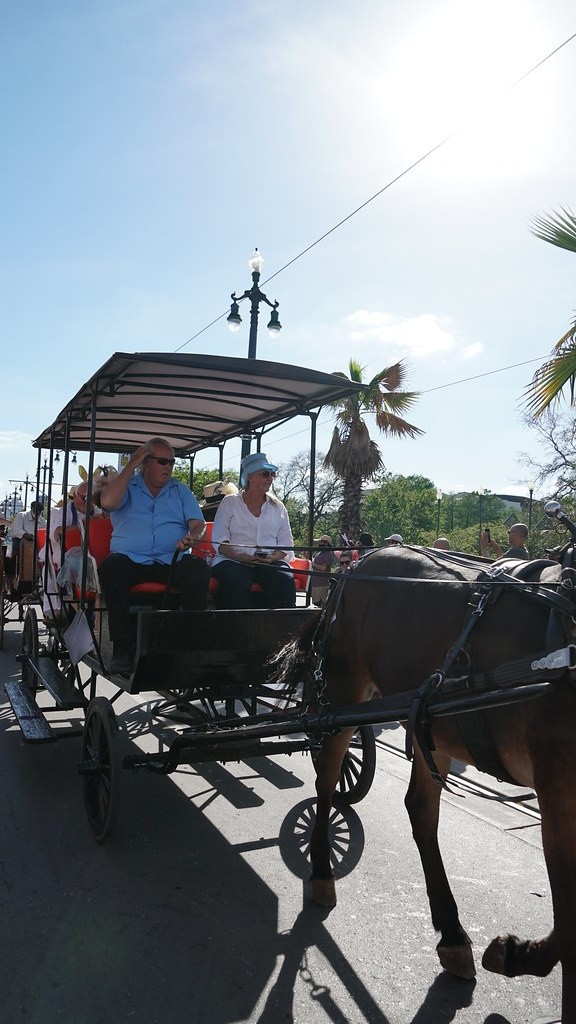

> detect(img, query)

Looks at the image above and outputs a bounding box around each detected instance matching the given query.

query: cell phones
[254,552,268,559]
[485,528,491,543]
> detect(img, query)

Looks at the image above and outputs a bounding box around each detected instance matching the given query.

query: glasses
[318,540,323,543]
[146,456,176,466]
[340,560,351,565]
[507,531,516,534]
[77,492,87,500]
[254,470,276,478]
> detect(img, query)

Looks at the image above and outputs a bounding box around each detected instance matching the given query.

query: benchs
[36,517,309,607]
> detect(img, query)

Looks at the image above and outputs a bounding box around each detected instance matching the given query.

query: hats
[544,545,563,554]
[360,533,374,545]
[317,535,332,547]
[32,500,45,510]
[242,453,278,478]
[384,533,403,543]
[202,481,239,508]
[433,538,450,550]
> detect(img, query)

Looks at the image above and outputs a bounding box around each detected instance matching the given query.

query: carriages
[0,351,575,1024]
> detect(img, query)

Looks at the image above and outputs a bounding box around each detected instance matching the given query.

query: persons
[309,534,334,608]
[336,532,404,576]
[39,471,118,619]
[0,501,48,595]
[479,523,529,560]
[85,438,211,674]
[211,453,296,609]
[433,537,449,550]
[544,545,563,563]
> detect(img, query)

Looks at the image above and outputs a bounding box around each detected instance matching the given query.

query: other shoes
[42,613,60,626]
[110,639,138,676]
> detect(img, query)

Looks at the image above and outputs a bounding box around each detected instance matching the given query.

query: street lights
[436,490,443,539]
[528,480,535,532]
[225,245,283,487]
[477,484,486,555]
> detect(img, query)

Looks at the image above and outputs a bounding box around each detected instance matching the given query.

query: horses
[280,542,576,979]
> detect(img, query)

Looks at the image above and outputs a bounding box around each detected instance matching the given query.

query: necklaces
[243,492,262,516]
